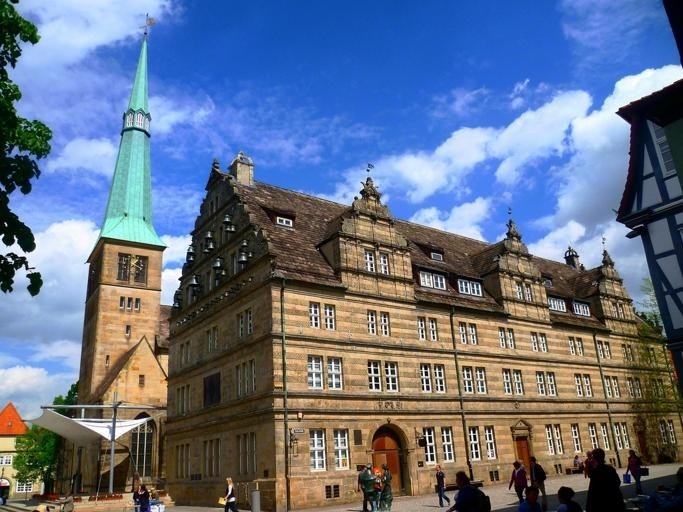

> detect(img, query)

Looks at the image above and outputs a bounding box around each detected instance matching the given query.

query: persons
[573,455,585,474]
[132,484,141,512]
[359,462,380,512]
[445,470,491,512]
[223,477,239,512]
[134,483,151,512]
[625,450,643,495]
[377,464,392,512]
[434,465,451,509]
[655,466,682,503]
[583,448,624,512]
[528,456,548,511]
[356,465,372,511]
[56,490,74,512]
[583,451,592,479]
[518,485,542,512]
[507,460,528,504]
[373,465,382,511]
[554,486,583,512]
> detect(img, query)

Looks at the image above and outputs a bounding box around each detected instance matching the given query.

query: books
[218,496,227,505]
[622,475,630,484]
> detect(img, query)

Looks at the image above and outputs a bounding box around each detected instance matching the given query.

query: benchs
[435,479,484,493]
[566,464,615,475]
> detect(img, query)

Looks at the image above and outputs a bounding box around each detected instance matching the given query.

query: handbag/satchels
[639,468,649,476]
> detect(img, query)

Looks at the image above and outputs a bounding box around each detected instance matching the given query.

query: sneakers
[438,499,450,508]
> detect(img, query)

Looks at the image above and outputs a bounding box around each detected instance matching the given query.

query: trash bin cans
[251,490,260,512]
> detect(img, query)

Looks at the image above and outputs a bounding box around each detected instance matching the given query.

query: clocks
[117,253,148,285]
